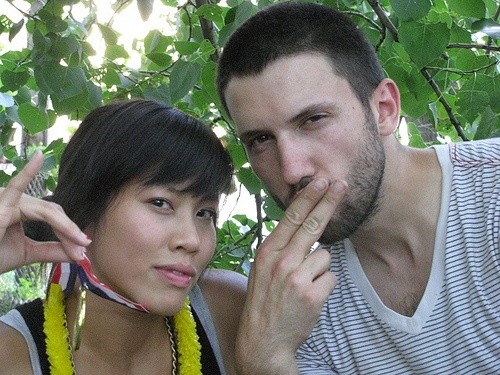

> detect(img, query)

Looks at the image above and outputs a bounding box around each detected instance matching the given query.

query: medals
[73,287,87,353]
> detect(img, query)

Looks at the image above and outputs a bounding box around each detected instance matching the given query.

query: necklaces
[62,298,176,375]
[43,280,204,375]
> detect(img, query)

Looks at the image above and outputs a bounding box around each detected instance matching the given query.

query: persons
[216,2,500,375]
[0,101,250,375]
